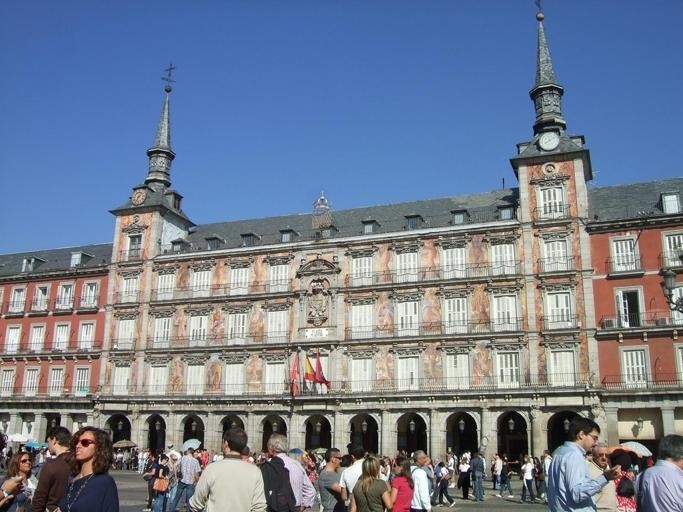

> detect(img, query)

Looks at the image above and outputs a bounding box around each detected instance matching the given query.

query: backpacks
[616,471,634,496]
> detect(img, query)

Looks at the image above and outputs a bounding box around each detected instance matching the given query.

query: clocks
[132,188,146,205]
[538,131,559,151]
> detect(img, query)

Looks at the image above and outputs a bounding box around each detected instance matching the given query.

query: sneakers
[495,494,535,504]
[432,493,484,508]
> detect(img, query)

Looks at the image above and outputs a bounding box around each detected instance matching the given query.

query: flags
[290,348,332,401]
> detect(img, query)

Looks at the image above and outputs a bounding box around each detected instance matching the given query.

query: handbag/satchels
[152,477,169,491]
[444,473,451,480]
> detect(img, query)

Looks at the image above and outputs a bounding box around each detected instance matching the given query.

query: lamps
[655,355,663,384]
[26,418,32,427]
[2,416,7,428]
[637,416,643,432]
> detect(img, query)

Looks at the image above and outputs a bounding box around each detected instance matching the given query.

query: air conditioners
[603,318,617,330]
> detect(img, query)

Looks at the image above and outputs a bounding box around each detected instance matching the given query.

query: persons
[452,418,683,511]
[0,425,119,511]
[111,427,458,512]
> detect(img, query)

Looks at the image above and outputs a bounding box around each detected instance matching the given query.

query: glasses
[587,433,597,441]
[20,458,31,463]
[335,456,342,459]
[75,438,95,447]
